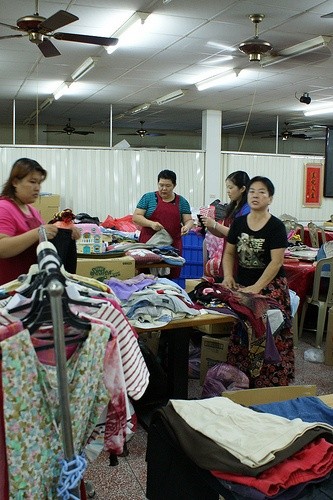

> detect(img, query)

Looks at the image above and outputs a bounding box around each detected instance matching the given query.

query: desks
[296,227,333,247]
[133,312,235,410]
[281,248,318,314]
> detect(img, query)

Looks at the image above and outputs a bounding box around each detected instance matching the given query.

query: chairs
[275,213,333,366]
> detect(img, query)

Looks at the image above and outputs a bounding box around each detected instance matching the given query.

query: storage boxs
[76,255,135,281]
[199,335,231,386]
[186,279,227,334]
[171,229,203,289]
[29,194,61,225]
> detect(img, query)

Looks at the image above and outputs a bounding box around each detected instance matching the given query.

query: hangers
[0,241,113,352]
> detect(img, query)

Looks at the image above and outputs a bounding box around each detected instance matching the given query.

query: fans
[117,120,167,136]
[0,0,119,58]
[194,13,320,64]
[42,117,95,136]
[261,121,312,141]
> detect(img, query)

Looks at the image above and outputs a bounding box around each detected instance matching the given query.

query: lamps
[39,11,333,120]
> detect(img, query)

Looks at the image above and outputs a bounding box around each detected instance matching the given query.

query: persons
[132,169,194,280]
[222,175,296,389]
[200,171,251,279]
[0,157,82,286]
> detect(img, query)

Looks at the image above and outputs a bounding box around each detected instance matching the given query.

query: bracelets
[213,222,218,229]
